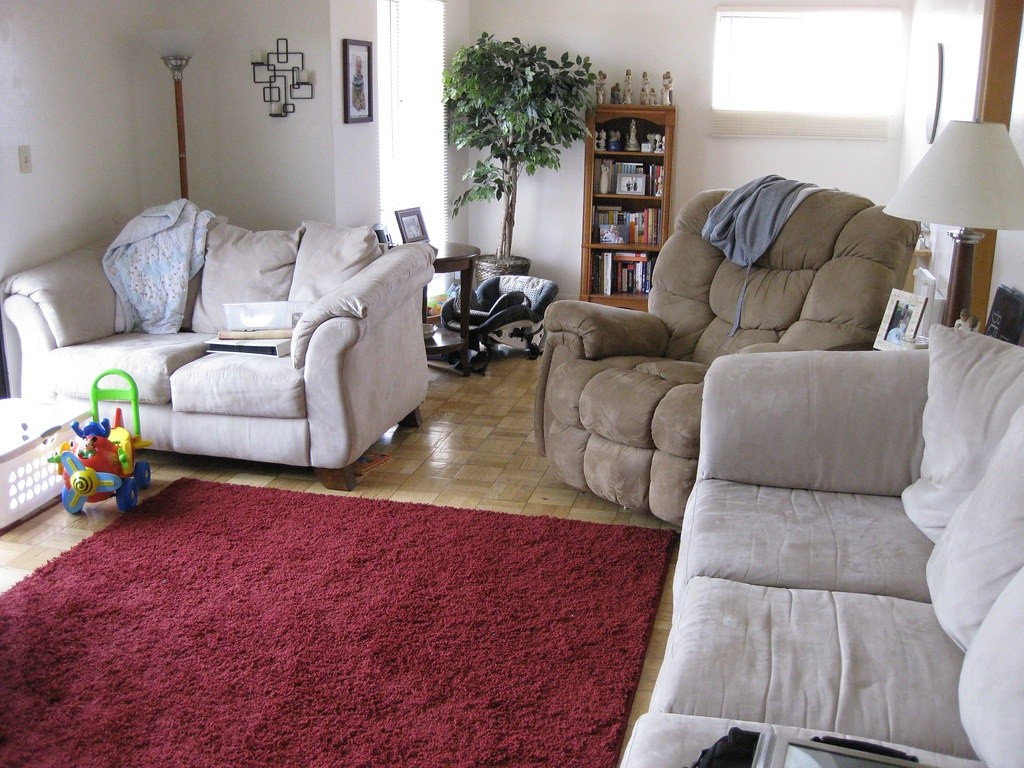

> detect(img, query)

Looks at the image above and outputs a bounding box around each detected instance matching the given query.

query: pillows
[287,220,380,309]
[188,224,309,334]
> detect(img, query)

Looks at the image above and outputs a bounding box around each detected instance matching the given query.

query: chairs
[440,276,557,354]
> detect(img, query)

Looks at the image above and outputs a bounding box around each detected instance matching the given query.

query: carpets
[0,475,676,768]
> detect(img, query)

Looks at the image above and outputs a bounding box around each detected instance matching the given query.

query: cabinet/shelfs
[395,206,426,243]
[578,101,677,312]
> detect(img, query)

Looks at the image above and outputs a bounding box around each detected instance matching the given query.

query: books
[592,206,661,245]
[594,158,663,196]
[590,250,657,296]
[205,339,291,357]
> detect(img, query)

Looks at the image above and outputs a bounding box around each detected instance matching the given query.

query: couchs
[6,216,436,492]
[620,324,1023,767]
[531,188,917,528]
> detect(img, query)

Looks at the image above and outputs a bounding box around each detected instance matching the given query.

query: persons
[602,225,616,242]
[600,159,609,194]
[885,304,912,345]
[352,56,366,111]
[595,69,674,105]
[595,119,665,154]
[954,309,978,331]
[632,179,638,192]
[625,180,631,192]
[611,229,625,243]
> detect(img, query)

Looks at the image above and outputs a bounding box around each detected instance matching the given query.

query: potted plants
[444,32,595,287]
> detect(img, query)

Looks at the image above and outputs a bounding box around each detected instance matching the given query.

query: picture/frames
[873,288,926,351]
[617,173,646,195]
[343,38,375,124]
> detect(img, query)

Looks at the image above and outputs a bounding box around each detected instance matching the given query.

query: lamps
[142,27,213,201]
[881,117,1024,345]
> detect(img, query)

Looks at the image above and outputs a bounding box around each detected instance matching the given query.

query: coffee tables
[1,395,87,533]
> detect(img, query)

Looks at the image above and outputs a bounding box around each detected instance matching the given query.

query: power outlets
[19,145,33,175]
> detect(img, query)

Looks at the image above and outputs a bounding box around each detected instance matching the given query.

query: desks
[400,242,481,374]
